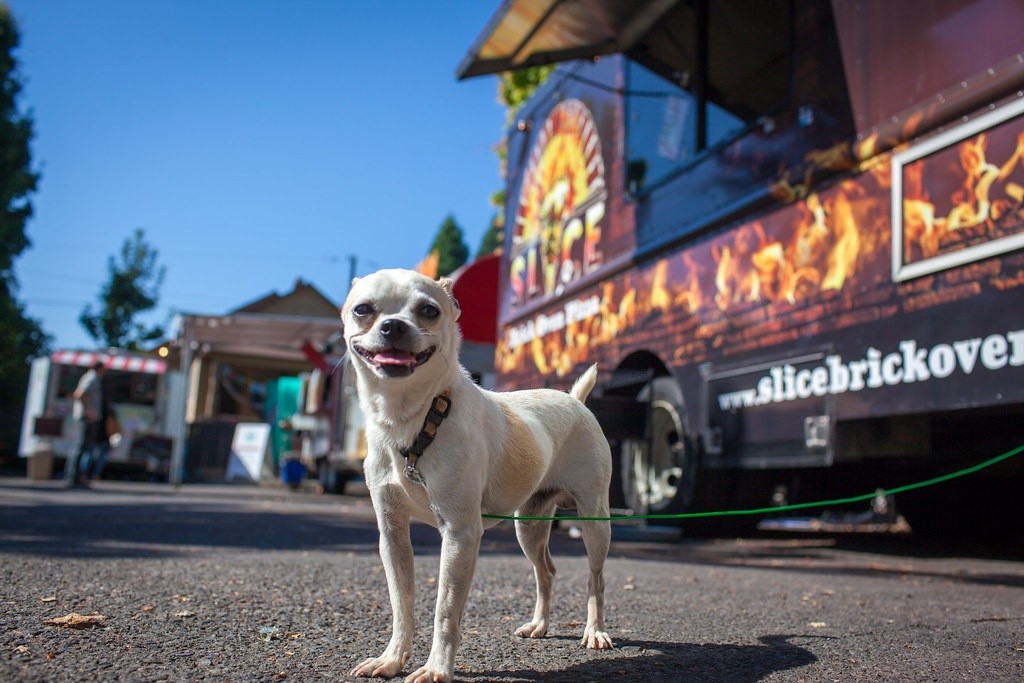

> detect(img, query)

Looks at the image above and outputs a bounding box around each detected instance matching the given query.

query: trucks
[277,0,1024,543]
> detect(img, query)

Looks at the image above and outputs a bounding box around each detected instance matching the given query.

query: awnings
[52,346,168,375]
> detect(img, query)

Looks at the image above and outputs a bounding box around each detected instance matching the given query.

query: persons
[62,363,122,492]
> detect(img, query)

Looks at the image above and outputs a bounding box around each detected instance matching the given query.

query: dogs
[339,266,616,683]
